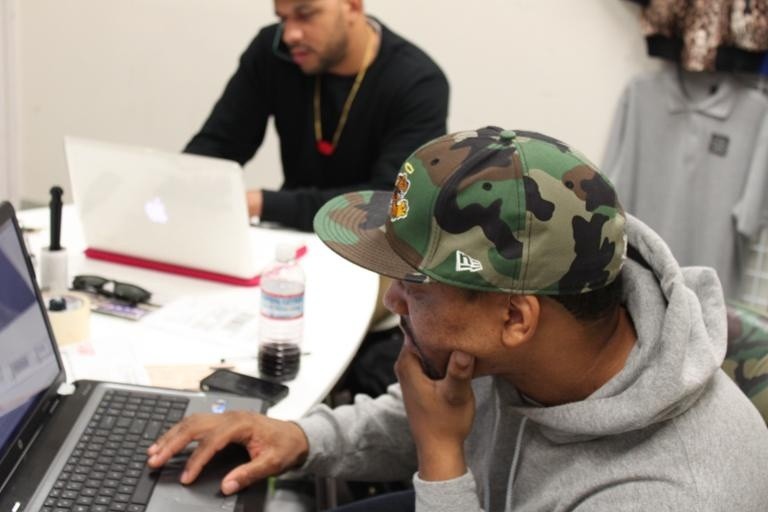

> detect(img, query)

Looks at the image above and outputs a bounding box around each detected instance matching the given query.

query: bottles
[258,241,306,384]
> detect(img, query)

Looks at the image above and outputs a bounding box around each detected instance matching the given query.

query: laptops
[0,201,269,512]
[63,137,305,280]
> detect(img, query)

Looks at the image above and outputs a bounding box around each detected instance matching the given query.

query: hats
[313,127,627,295]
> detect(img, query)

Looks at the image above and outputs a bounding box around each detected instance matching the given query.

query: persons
[146,125,768,512]
[180,0,449,230]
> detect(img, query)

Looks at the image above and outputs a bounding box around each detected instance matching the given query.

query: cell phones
[199,369,289,407]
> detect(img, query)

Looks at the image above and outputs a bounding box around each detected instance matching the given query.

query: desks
[8,204,394,512]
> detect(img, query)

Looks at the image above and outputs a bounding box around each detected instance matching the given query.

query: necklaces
[314,28,375,154]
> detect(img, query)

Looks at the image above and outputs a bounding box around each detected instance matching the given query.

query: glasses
[69,275,151,308]
